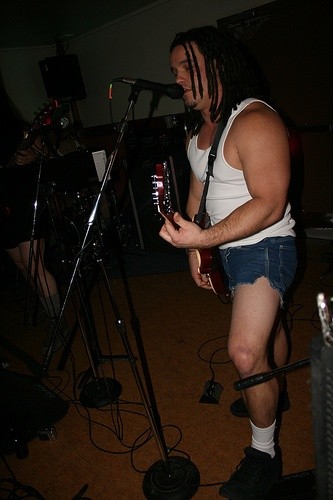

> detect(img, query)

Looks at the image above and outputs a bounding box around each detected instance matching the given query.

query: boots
[40,292,69,353]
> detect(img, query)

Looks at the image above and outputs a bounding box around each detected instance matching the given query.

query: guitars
[151,161,229,295]
[0,99,69,215]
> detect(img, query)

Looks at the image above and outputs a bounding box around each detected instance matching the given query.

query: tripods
[76,268,133,390]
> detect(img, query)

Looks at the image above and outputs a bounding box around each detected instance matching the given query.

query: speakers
[39,53,87,101]
[0,366,68,459]
[124,135,191,255]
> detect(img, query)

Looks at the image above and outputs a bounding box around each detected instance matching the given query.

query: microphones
[113,77,184,100]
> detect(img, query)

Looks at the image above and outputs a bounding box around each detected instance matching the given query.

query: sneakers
[230,388,290,417]
[219,446,282,500]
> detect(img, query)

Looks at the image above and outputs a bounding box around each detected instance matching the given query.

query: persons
[158,25,297,500]
[0,84,60,319]
